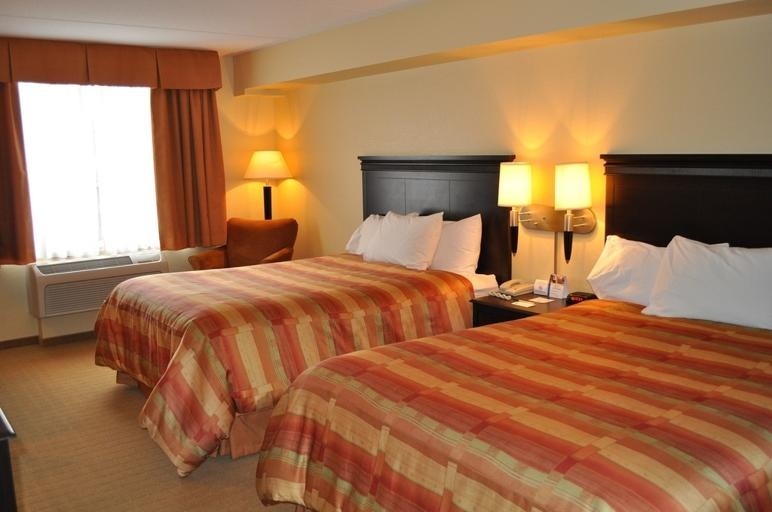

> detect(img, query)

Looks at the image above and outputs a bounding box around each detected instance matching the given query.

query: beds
[256,154,772,512]
[95,155,515,477]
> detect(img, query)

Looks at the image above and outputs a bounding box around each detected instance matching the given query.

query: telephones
[488,279,534,300]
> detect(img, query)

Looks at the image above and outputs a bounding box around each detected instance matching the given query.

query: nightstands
[469,293,570,327]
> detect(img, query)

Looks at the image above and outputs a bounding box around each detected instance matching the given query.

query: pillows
[363,211,444,271]
[345,213,419,256]
[641,235,772,330]
[586,235,730,307]
[429,214,482,273]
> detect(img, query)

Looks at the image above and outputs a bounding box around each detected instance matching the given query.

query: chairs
[188,218,298,270]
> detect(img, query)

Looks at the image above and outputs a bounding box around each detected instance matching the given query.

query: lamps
[497,161,597,263]
[243,150,294,220]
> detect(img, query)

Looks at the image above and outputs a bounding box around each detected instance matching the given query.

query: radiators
[26,249,169,344]
